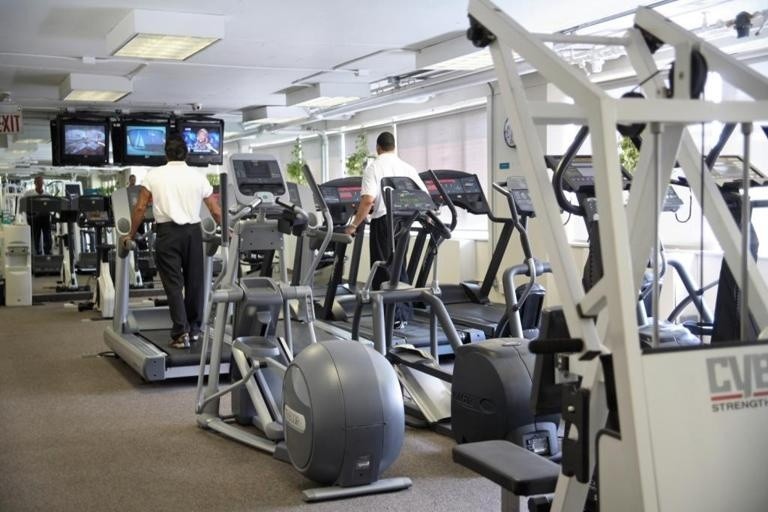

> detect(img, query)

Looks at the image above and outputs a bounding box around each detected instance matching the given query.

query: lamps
[57,9,224,103]
[241,37,519,124]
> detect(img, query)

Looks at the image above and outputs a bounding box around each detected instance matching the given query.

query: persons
[125,174,147,259]
[119,135,233,349]
[344,131,435,332]
[189,128,218,155]
[21,175,55,257]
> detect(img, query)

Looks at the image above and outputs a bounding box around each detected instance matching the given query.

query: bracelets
[350,223,357,227]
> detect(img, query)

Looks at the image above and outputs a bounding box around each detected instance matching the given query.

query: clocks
[503,117,516,148]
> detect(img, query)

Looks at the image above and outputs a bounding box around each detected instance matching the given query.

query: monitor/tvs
[177,117,224,165]
[112,121,169,166]
[64,183,82,197]
[51,119,110,168]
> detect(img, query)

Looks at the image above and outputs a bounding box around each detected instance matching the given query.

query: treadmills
[313,175,489,361]
[418,169,508,333]
[228,158,370,353]
[21,196,98,277]
[104,183,233,383]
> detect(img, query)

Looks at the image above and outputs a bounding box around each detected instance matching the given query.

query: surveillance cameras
[192,104,203,112]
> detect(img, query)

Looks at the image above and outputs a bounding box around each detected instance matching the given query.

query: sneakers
[191,332,201,341]
[393,320,408,330]
[167,333,191,349]
